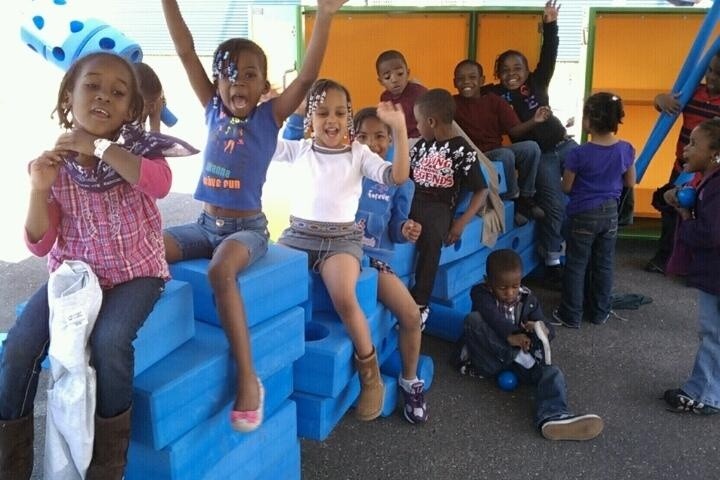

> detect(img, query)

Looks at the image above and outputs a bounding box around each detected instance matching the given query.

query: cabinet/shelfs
[581,6,720,243]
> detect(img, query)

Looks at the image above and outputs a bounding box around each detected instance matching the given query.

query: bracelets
[92,137,119,161]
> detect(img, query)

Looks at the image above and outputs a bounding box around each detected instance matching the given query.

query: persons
[465,250,604,441]
[258,50,551,424]
[643,49,720,272]
[552,94,638,328]
[158,2,348,433]
[483,1,598,277]
[0,51,173,478]
[651,116,720,413]
[132,62,163,151]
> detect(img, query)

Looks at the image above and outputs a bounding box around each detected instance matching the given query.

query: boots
[85,404,132,480]
[354,344,385,421]
[1,416,34,480]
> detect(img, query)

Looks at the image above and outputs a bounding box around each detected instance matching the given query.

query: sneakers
[528,321,552,366]
[514,212,531,226]
[231,378,265,431]
[664,388,718,416]
[540,413,604,442]
[419,305,430,332]
[553,306,580,328]
[399,374,429,424]
[516,196,545,220]
